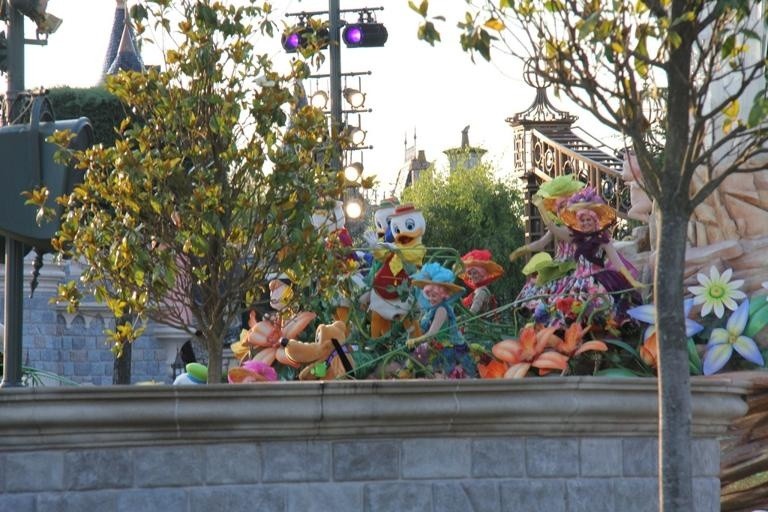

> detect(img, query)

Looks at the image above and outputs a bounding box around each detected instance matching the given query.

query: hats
[412,279,465,292]
[453,260,503,278]
[543,194,572,212]
[560,203,616,233]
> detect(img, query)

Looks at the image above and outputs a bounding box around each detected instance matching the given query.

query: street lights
[282,1,390,234]
[0,0,65,389]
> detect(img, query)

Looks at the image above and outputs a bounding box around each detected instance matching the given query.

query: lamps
[343,24,388,47]
[311,87,364,219]
[281,27,329,53]
[37,13,63,40]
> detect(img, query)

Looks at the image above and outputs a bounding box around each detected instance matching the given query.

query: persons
[509,173,586,319]
[404,263,481,377]
[451,249,504,322]
[531,188,646,330]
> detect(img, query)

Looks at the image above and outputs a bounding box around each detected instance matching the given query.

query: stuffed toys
[353,207,396,336]
[310,200,368,351]
[173,361,207,386]
[362,205,426,340]
[279,320,356,381]
